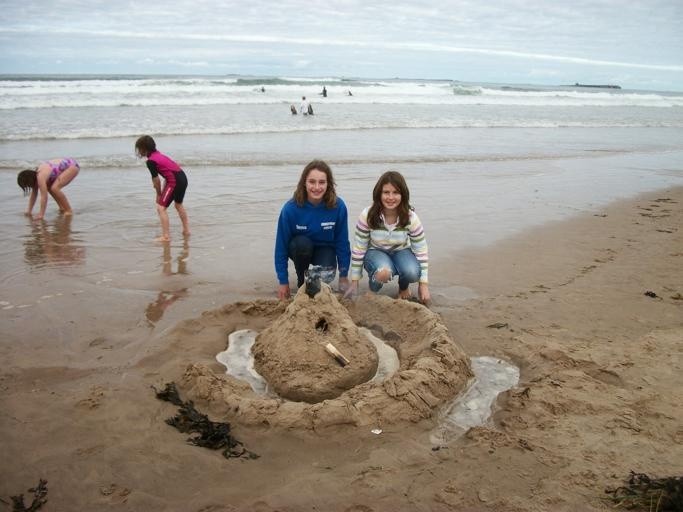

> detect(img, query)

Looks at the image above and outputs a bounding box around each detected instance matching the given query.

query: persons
[339,171,430,304]
[274,159,349,303]
[290,105,296,114]
[134,135,189,241]
[298,96,310,115]
[16,157,80,219]
[307,104,312,114]
[322,86,327,97]
